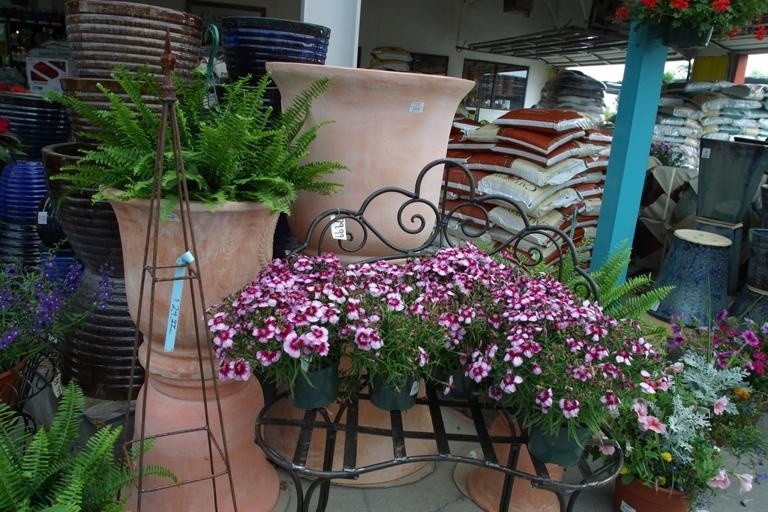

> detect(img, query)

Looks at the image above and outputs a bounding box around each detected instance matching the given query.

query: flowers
[612,0,768,40]
[623,306,768,504]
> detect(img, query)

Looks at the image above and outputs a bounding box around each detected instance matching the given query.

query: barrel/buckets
[647,228,734,329]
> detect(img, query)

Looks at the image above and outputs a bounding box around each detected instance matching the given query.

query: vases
[263,61,478,264]
[662,16,698,47]
[646,137,767,332]
[617,477,696,512]
